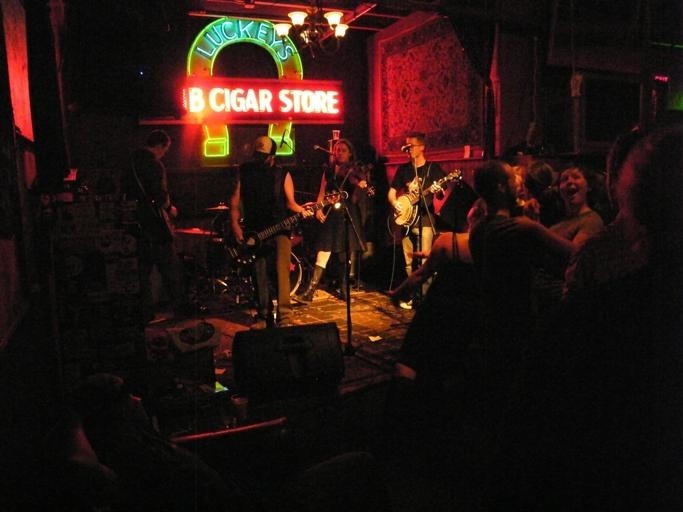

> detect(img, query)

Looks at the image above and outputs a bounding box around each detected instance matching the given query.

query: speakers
[232,322,345,397]
[358,247,408,288]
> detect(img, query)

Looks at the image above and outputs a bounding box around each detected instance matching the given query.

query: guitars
[221,190,348,267]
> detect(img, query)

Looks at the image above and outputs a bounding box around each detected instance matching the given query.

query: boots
[292,264,325,305]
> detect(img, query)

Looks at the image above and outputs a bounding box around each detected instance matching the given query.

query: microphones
[314,145,330,154]
[279,129,286,147]
[400,144,411,151]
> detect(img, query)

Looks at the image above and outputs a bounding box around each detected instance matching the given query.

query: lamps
[273,0,349,63]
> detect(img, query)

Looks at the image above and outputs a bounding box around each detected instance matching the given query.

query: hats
[253,135,277,156]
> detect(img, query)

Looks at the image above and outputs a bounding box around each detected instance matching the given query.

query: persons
[388,116,683,510]
[2,330,392,510]
[117,130,177,327]
[292,138,374,304]
[387,133,448,309]
[228,136,317,328]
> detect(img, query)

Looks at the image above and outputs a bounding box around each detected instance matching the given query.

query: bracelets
[164,203,171,212]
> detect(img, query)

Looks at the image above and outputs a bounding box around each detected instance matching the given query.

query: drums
[268,251,303,298]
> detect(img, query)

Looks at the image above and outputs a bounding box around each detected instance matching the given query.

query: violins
[335,163,377,198]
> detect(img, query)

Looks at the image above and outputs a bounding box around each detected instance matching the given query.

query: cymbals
[174,228,218,236]
[204,205,229,211]
